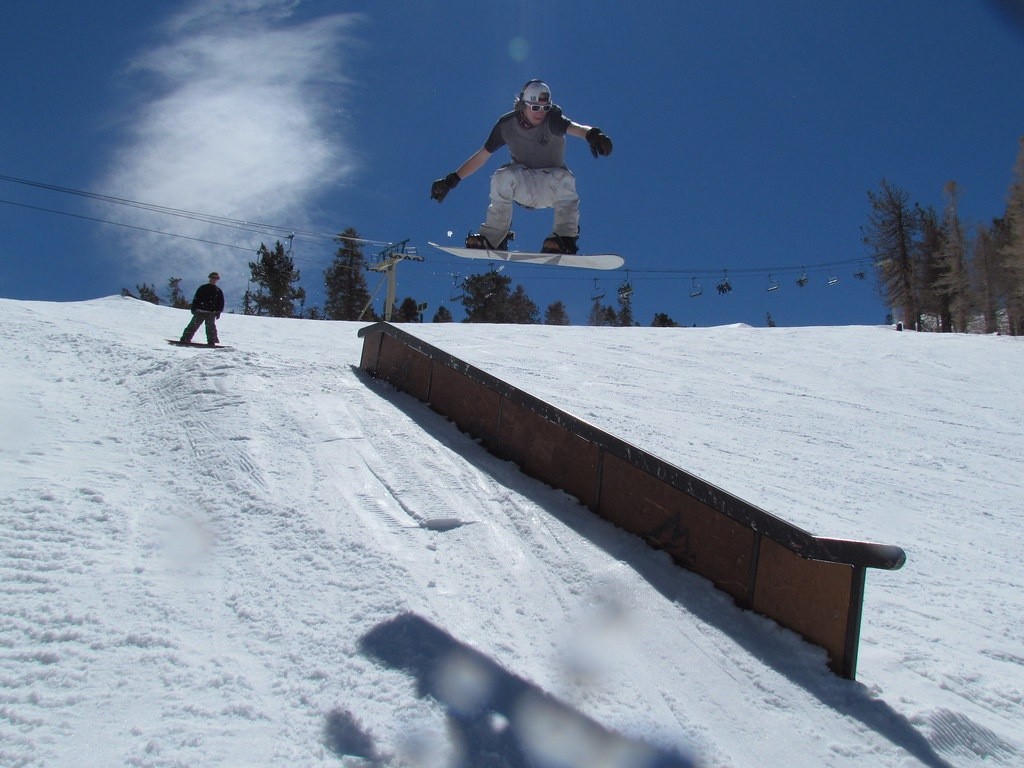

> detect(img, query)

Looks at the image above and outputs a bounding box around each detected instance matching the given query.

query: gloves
[214,311,220,319]
[585,127,613,160]
[431,172,462,204]
[190,307,196,314]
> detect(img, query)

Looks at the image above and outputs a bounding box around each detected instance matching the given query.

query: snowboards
[427,240,624,270]
[164,338,232,349]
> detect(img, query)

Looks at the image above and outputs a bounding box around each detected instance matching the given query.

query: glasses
[209,275,219,279]
[524,102,552,113]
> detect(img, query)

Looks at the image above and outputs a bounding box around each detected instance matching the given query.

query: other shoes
[180,337,191,343]
[540,236,579,255]
[207,342,215,346]
[465,230,515,251]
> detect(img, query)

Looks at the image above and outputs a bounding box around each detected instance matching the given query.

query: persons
[430,79,614,255]
[180,272,224,345]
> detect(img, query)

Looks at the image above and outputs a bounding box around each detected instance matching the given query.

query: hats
[519,79,551,103]
